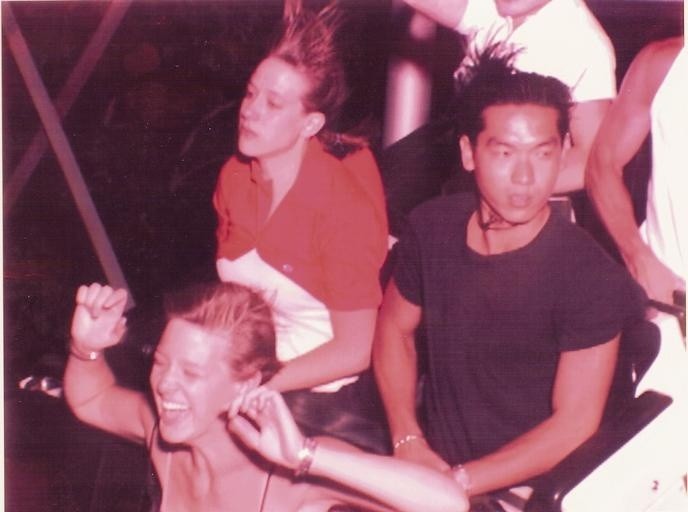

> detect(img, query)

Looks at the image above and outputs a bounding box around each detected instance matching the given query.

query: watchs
[451,463,471,493]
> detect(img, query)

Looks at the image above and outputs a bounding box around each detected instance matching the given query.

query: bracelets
[67,341,103,362]
[293,436,317,480]
[393,435,416,448]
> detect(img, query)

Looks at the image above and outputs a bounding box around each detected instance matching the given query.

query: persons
[402,1,618,193]
[580,36,685,314]
[370,73,625,494]
[210,0,388,393]
[60,281,471,512]
